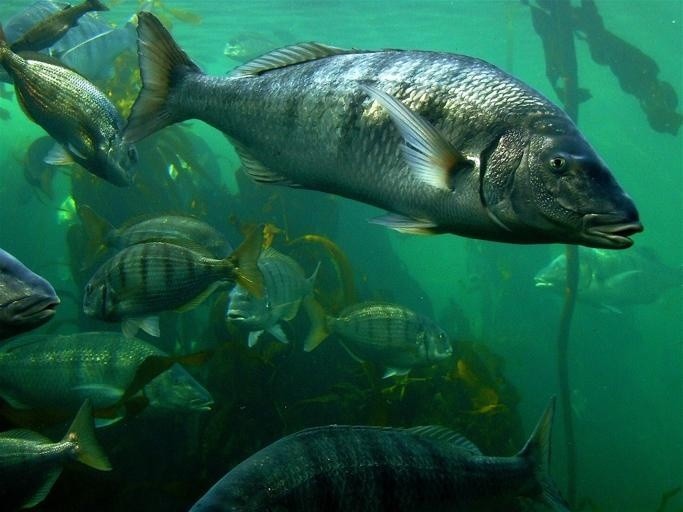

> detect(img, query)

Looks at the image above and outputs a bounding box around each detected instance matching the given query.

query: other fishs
[1,242,569,512]
[0,1,278,260]
